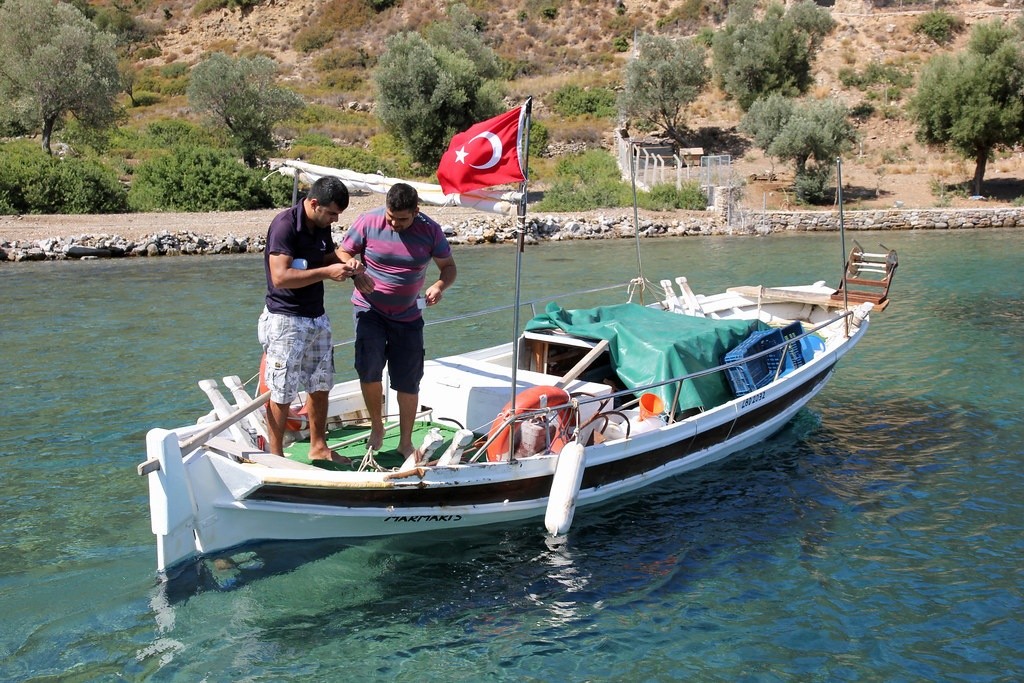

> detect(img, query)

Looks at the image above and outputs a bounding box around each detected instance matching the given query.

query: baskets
[720,322,814,398]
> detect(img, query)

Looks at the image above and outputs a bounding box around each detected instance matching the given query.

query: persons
[257,176,366,465]
[337,183,457,458]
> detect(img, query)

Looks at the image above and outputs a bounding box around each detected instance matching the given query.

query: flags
[437,106,526,195]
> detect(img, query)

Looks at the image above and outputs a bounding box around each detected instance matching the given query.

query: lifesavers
[486,385,573,463]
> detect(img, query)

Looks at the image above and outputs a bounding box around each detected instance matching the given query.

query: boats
[136,236,899,574]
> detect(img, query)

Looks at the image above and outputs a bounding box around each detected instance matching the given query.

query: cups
[416,298,428,309]
